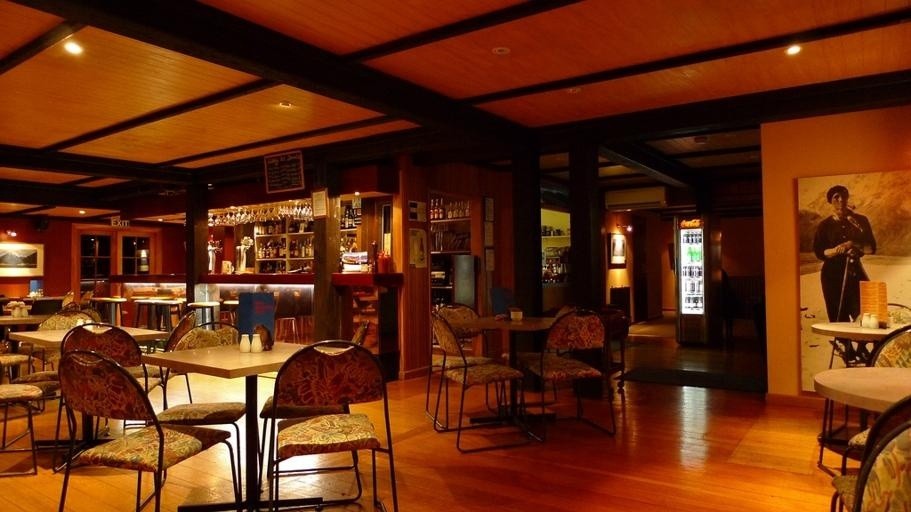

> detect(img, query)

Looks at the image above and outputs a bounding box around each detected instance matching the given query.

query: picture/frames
[1,243,45,278]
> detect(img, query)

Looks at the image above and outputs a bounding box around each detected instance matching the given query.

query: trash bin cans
[237,292,274,350]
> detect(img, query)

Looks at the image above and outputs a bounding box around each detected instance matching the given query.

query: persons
[813,185,877,365]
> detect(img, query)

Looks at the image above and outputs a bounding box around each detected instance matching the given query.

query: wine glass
[208,199,313,227]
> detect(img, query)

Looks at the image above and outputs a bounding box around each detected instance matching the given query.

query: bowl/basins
[510,312,522,321]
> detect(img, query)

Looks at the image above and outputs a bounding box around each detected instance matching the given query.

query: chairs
[0,295,244,492]
[268,340,400,511]
[57,348,238,511]
[809,302,911,511]
[428,297,631,453]
[260,317,373,489]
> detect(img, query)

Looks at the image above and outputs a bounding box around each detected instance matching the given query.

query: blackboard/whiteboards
[264,150,305,193]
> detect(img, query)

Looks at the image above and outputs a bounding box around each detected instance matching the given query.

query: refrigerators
[674,216,724,350]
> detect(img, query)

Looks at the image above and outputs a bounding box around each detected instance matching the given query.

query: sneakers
[845,349,853,363]
[856,348,869,360]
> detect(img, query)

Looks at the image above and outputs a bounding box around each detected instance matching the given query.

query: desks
[139,340,322,511]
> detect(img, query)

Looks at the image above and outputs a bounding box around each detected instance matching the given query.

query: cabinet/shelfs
[427,218,473,255]
[254,207,368,274]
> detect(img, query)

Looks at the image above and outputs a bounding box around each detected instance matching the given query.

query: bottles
[14,291,41,319]
[862,312,879,329]
[341,207,357,229]
[542,262,557,277]
[261,261,272,272]
[681,261,704,308]
[238,333,262,354]
[258,223,281,236]
[430,197,471,220]
[76,319,94,333]
[681,229,701,243]
[258,236,346,259]
